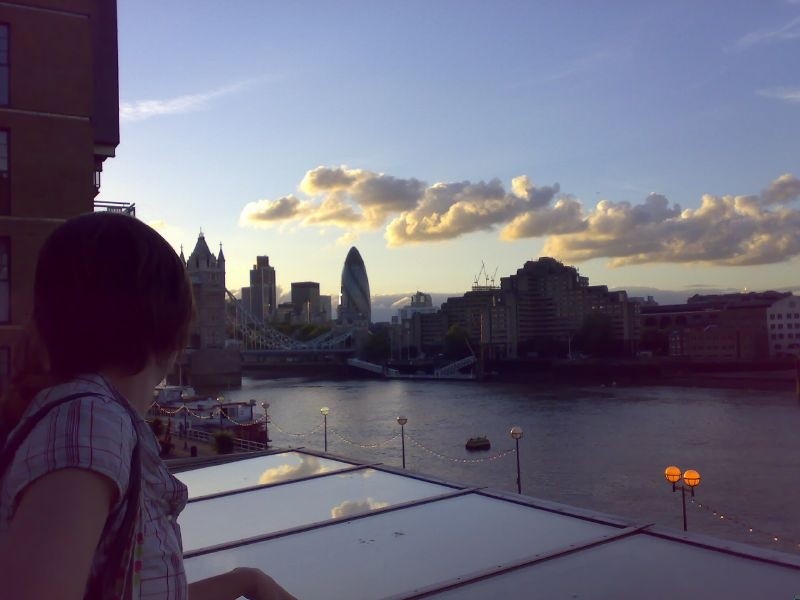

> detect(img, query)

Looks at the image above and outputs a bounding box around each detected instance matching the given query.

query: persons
[178,421,184,440]
[0,211,297,600]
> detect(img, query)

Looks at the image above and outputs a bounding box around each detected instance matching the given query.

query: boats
[465,437,492,450]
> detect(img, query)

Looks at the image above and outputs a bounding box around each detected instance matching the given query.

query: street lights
[396,415,408,468]
[261,400,271,449]
[180,391,190,443]
[153,390,160,423]
[248,398,257,422]
[510,426,525,496]
[216,396,225,427]
[664,465,701,531]
[320,404,330,452]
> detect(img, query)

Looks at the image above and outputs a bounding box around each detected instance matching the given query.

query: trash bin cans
[190,445,197,457]
